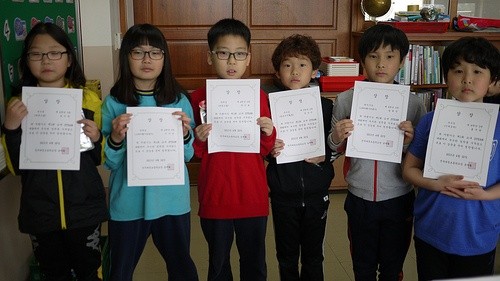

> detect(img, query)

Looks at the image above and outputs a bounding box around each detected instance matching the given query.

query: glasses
[27,50,67,61]
[129,49,164,60]
[212,50,248,61]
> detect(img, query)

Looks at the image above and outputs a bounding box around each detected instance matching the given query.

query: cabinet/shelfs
[353,31,500,115]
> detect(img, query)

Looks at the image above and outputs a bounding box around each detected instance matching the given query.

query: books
[392,44,457,112]
[393,8,441,22]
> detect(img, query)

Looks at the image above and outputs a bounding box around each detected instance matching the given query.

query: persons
[402,36,500,281]
[327,23,427,281]
[3,22,106,281]
[102,24,199,281]
[261,35,345,281]
[182,18,276,281]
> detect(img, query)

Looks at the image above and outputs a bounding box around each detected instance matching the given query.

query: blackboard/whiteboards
[0,0,85,140]
